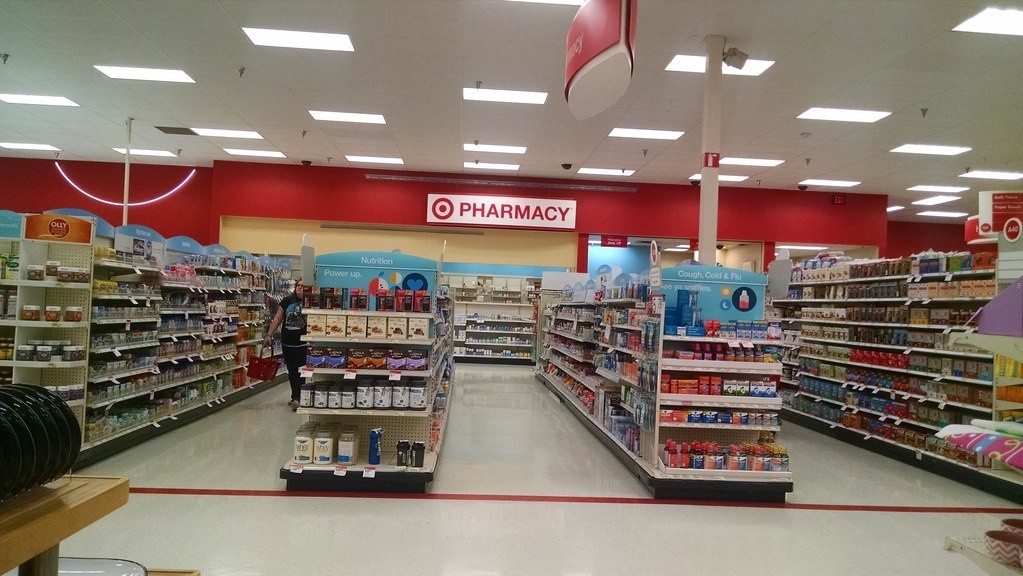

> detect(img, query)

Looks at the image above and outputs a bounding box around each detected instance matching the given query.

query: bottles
[665,430,788,472]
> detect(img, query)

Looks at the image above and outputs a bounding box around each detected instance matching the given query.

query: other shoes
[288,400,300,410]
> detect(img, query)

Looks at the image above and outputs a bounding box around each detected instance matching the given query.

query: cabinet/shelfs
[1,244,1021,505]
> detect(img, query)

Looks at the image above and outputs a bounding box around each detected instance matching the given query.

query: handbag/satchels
[246,342,279,382]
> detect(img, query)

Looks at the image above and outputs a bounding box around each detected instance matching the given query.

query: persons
[262,275,309,410]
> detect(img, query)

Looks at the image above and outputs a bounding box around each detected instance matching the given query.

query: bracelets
[266,333,274,340]
